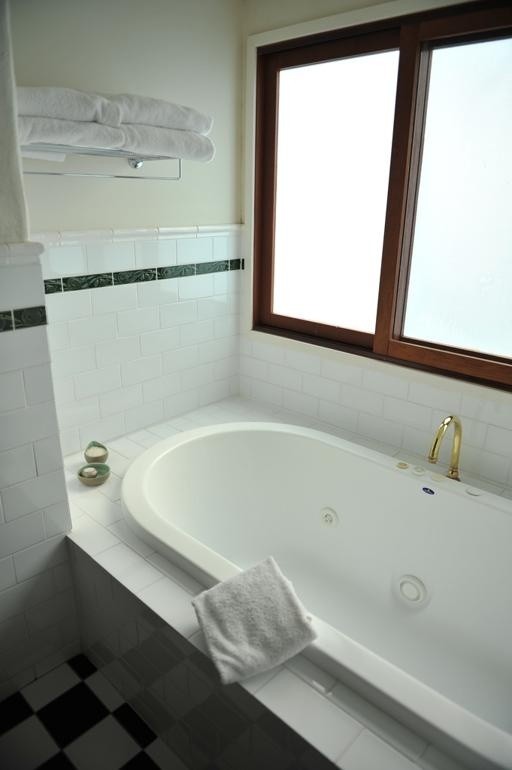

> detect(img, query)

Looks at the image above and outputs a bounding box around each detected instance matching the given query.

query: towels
[193,555,323,686]
[14,82,218,160]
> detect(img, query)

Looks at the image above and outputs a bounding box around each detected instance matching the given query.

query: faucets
[428,414,461,479]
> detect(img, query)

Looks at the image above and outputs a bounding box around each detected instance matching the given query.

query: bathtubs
[117,420,512,770]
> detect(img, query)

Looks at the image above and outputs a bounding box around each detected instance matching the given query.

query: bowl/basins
[77,440,110,486]
